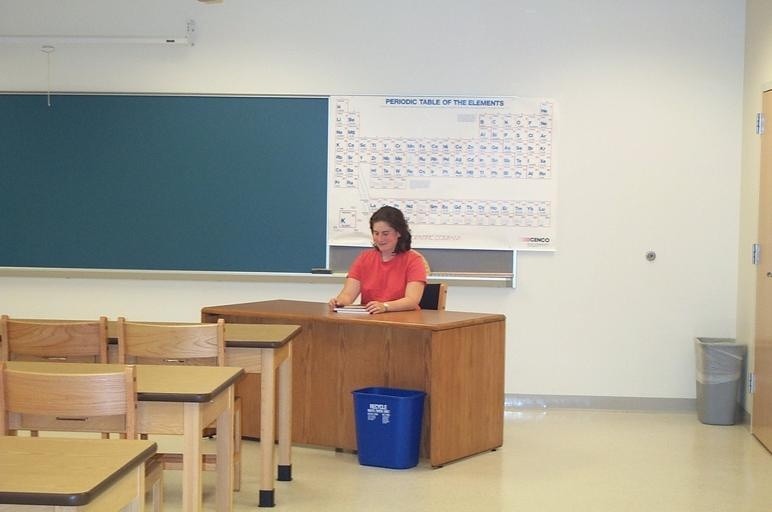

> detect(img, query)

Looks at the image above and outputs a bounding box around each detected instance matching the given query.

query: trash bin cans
[383,303,388,312]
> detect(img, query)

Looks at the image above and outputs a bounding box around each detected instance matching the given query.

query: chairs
[420,284,448,309]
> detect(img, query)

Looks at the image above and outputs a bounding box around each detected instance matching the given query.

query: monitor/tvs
[334,305,370,314]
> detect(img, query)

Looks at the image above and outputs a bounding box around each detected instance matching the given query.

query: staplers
[311,268,333,274]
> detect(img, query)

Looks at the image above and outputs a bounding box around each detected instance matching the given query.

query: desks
[197,298,507,465]
[2,313,306,512]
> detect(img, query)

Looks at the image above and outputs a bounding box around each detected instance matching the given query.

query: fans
[329,245,516,280]
[0,91,329,278]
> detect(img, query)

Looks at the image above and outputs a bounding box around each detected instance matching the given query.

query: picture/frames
[197,298,507,465]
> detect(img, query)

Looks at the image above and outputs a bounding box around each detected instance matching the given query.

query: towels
[695,337,746,426]
[350,387,427,469]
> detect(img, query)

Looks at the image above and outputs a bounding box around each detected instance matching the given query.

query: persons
[329,207,431,315]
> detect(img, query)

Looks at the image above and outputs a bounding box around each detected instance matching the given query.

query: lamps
[420,284,448,309]
